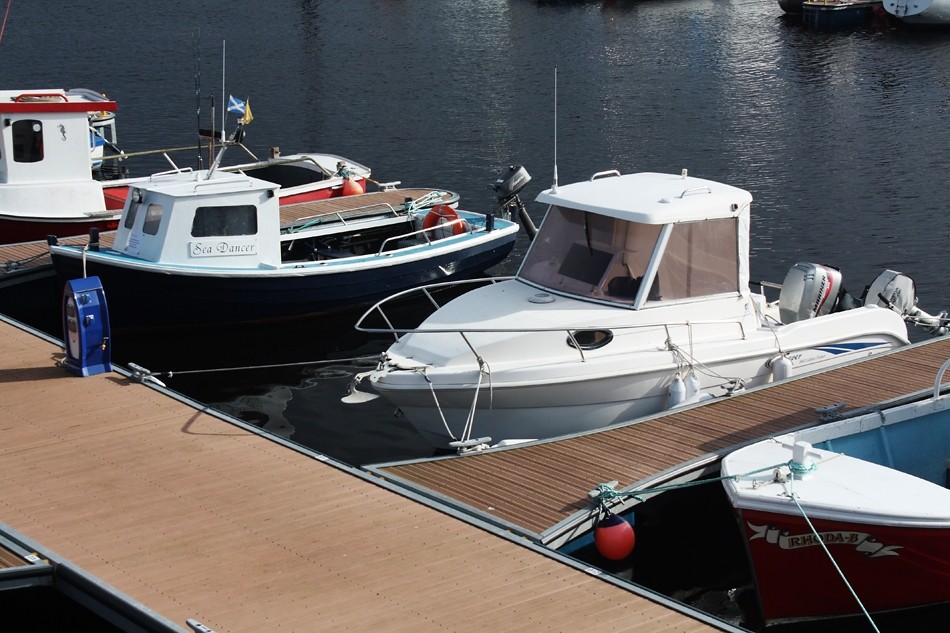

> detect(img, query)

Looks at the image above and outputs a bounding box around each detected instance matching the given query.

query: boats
[47,40,541,366]
[0,89,373,245]
[341,66,950,459]
[777,0,950,26]
[720,359,950,633]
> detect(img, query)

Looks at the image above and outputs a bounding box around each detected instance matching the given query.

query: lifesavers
[423,206,463,239]
